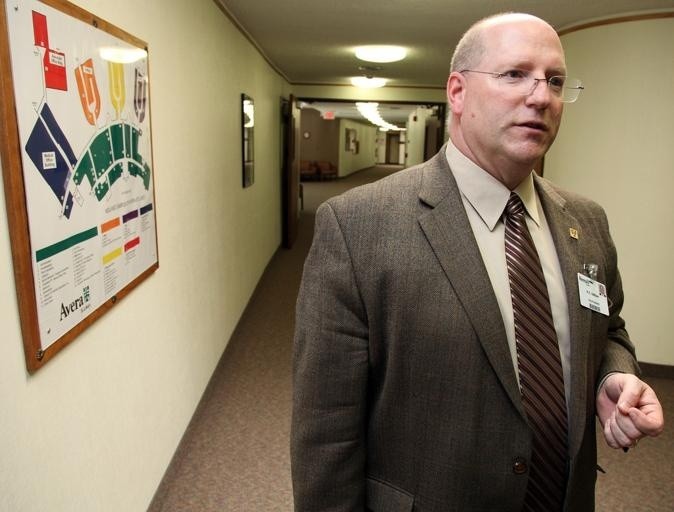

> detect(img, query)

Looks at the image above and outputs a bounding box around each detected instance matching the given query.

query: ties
[504,191,570,512]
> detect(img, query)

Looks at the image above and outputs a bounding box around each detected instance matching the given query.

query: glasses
[459,69,584,103]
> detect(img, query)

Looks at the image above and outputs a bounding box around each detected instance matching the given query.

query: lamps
[349,64,388,88]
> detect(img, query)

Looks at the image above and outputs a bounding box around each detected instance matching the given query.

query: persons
[289,12,665,512]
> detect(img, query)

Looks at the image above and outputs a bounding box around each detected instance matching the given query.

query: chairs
[299,161,337,181]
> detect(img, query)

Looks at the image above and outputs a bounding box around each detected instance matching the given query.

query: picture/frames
[241,92,258,187]
[0,1,162,375]
